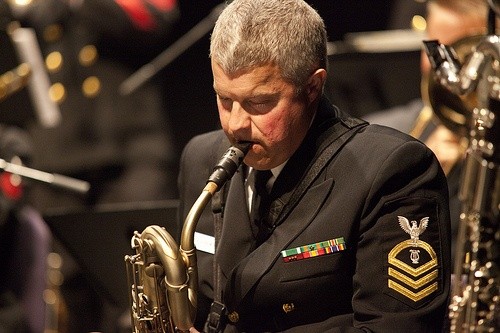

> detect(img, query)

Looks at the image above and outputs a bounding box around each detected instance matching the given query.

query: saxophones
[122,139,255,333]
[416,35,500,333]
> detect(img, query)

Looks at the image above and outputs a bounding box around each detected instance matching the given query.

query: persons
[175,0,454,333]
[0,0,181,333]
[357,0,500,303]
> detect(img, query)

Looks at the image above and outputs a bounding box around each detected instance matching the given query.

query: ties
[249,169,273,254]
